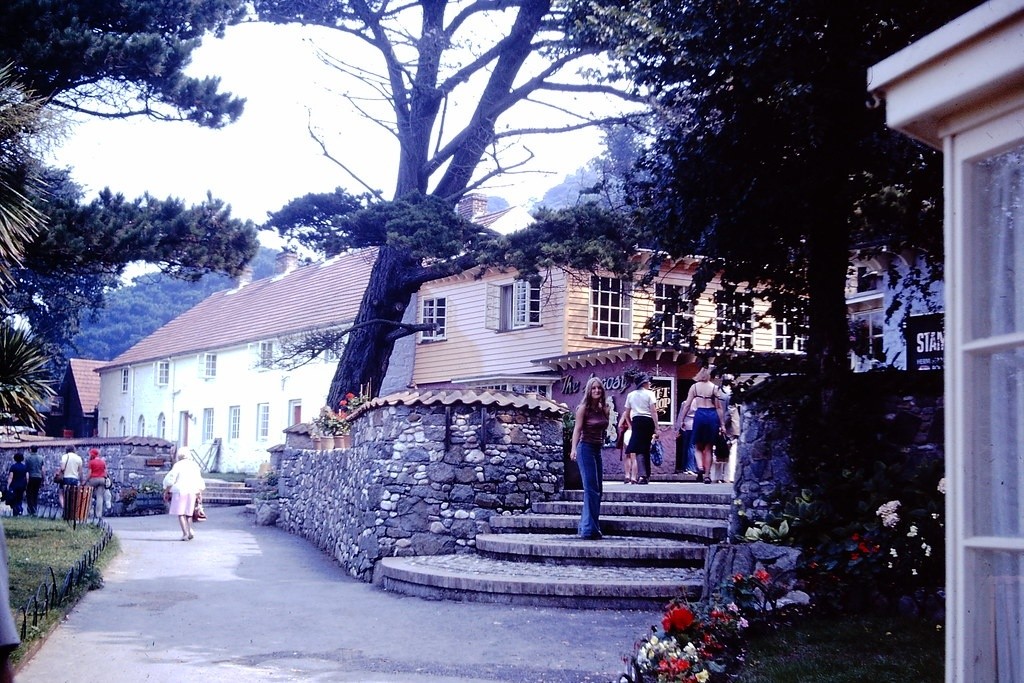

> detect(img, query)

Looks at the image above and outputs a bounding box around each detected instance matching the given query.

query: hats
[90,449,99,458]
[635,372,653,389]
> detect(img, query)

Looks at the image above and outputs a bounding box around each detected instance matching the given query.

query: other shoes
[631,480,637,484]
[583,529,603,539]
[624,477,629,482]
[638,476,650,484]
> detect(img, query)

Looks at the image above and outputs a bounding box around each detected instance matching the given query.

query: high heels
[180,535,188,541]
[188,532,194,539]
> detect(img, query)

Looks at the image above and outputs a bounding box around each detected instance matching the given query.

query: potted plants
[308,404,352,450]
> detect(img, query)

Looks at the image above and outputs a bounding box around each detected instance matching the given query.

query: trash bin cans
[64,484,93,520]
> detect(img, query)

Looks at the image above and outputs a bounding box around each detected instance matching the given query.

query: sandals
[696,471,705,481]
[703,477,712,483]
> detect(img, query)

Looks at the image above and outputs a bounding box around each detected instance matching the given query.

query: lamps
[187,414,196,425]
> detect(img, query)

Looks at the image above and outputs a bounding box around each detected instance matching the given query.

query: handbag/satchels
[54,469,64,483]
[193,498,207,522]
[650,438,664,467]
[715,431,731,462]
[105,475,112,488]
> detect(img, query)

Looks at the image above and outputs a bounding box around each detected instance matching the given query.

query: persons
[679,368,726,484]
[624,371,662,485]
[7,452,29,516]
[0,524,21,683]
[675,398,698,476]
[83,448,107,521]
[571,377,609,538]
[619,410,639,483]
[57,444,83,509]
[25,445,45,516]
[162,447,205,541]
[715,373,740,483]
